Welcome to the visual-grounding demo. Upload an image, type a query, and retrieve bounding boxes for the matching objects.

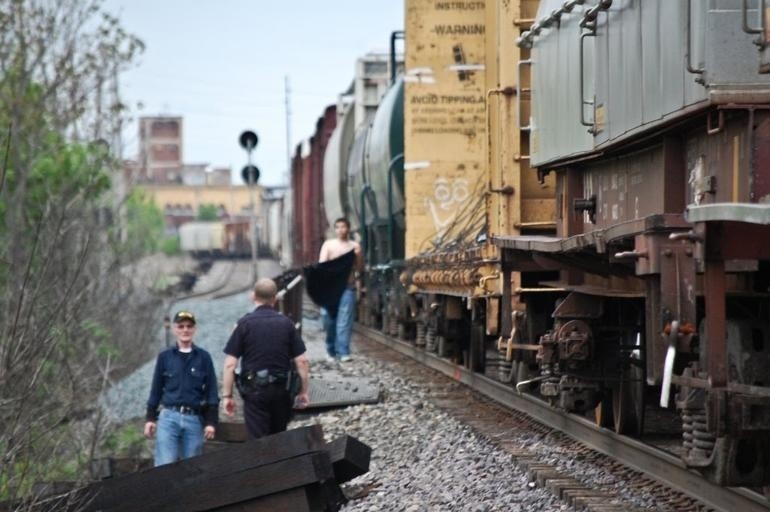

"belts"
[168,405,198,415]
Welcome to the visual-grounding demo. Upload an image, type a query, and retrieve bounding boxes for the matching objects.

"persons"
[317,217,362,363]
[221,277,310,441]
[144,311,220,468]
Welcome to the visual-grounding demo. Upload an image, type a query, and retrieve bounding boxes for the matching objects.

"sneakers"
[341,356,353,361]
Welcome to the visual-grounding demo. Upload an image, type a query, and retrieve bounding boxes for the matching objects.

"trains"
[177,0,769,486]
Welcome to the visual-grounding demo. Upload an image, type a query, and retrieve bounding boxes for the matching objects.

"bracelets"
[222,395,234,398]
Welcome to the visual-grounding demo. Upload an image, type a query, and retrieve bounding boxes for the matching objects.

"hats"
[173,311,195,324]
